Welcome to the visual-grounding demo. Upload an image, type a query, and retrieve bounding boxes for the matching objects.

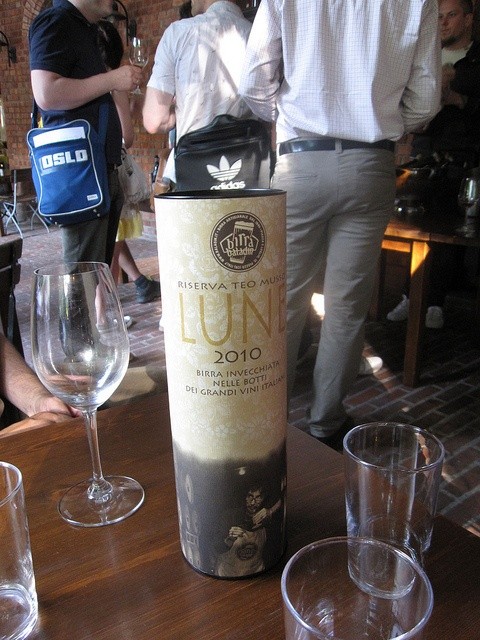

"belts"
[280,139,390,156]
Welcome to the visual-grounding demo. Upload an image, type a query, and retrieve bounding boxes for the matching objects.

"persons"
[27,0,146,372]
[385,1,478,332]
[0,321,84,438]
[142,0,272,189]
[239,1,443,447]
[111,240,160,304]
[90,20,146,335]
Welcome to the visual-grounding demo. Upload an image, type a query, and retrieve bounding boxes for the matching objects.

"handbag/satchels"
[394,152,466,233]
[173,114,271,191]
[26,8,112,227]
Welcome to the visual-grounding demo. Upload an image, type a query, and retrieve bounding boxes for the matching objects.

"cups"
[282,536,435,640]
[342,422,445,598]
[1,461,41,639]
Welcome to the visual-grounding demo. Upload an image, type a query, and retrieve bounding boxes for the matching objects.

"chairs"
[1,167,51,240]
[0,175,19,223]
[1,237,26,373]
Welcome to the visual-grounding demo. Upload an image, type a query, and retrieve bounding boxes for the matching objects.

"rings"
[136,79,141,85]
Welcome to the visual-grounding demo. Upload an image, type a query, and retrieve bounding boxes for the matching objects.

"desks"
[1,389,480,640]
[383,215,480,385]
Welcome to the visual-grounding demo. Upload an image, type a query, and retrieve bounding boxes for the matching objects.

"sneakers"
[359,346,383,376]
[386,294,410,321]
[96,316,133,334]
[136,280,160,304]
[426,306,444,329]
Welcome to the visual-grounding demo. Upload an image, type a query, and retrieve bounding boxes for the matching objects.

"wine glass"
[128,36,151,96]
[31,260,146,529]
[455,176,480,238]
